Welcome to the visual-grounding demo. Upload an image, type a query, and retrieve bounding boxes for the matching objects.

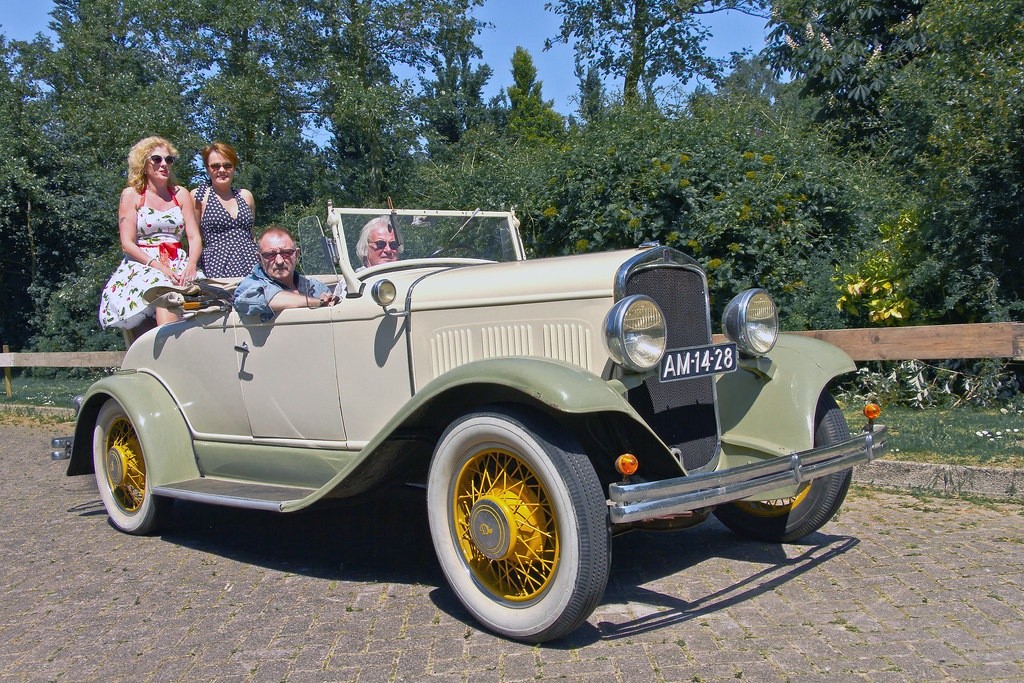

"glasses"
[369,240,399,250]
[146,155,176,164]
[260,249,295,259]
[208,162,234,170]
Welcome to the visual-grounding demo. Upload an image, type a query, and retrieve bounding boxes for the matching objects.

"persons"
[98,135,208,327]
[232,226,335,322]
[188,142,261,280]
[333,216,404,305]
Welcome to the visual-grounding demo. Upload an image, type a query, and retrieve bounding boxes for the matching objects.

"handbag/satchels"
[142,276,245,316]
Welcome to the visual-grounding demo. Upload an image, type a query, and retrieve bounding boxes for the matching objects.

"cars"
[50,199,898,644]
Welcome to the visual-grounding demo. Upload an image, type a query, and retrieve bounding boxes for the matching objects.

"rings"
[171,277,176,282]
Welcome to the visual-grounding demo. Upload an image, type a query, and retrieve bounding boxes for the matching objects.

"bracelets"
[145,258,157,269]
[317,297,326,308]
